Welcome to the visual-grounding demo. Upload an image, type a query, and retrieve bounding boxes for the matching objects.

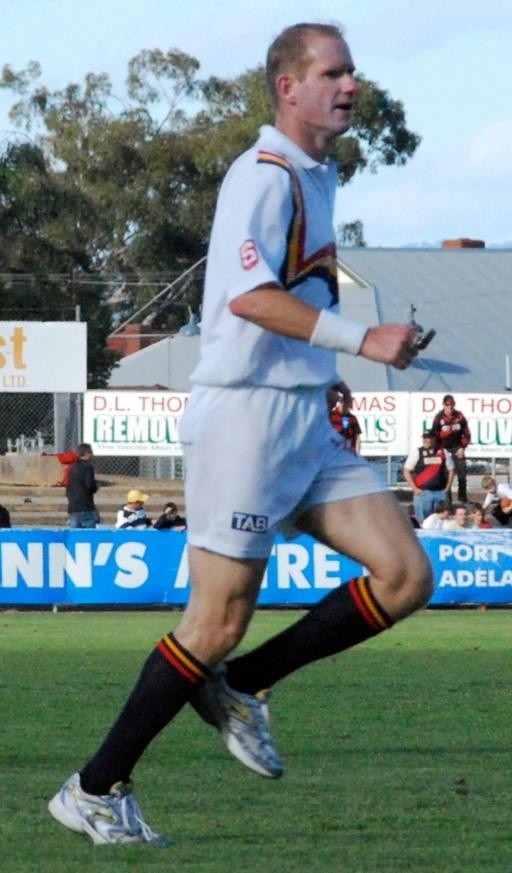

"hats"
[127,490,149,503]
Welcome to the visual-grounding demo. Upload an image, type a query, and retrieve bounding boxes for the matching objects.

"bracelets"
[306,305,371,358]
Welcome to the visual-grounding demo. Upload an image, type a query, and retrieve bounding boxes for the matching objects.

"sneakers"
[48,773,166,845]
[188,661,283,778]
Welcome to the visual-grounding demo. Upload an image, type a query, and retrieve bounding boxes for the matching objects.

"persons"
[421,499,493,529]
[430,395,471,504]
[480,476,512,526]
[115,490,154,530]
[329,394,362,454]
[404,430,456,524]
[45,21,434,850]
[65,445,100,530]
[154,502,187,531]
